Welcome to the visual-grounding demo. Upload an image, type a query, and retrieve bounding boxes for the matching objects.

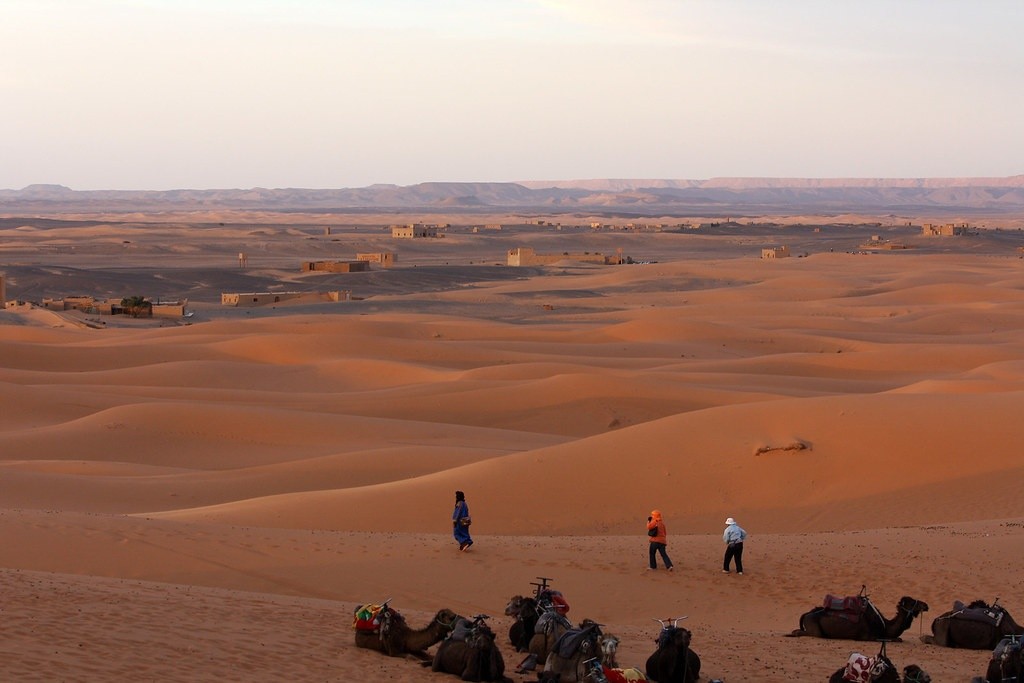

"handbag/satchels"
[647,520,658,536]
[460,517,471,526]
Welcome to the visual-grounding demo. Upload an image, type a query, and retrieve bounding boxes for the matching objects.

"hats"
[725,518,735,524]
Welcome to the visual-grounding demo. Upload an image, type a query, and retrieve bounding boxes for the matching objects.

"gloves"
[648,516,652,521]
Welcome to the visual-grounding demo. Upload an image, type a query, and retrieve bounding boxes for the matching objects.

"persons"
[647,510,673,572]
[722,518,747,575]
[452,491,473,551]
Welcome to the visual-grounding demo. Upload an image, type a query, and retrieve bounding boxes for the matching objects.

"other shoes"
[465,541,473,551]
[667,566,673,571]
[722,570,729,573]
[647,568,656,570]
[736,572,742,575]
[459,542,468,552]
